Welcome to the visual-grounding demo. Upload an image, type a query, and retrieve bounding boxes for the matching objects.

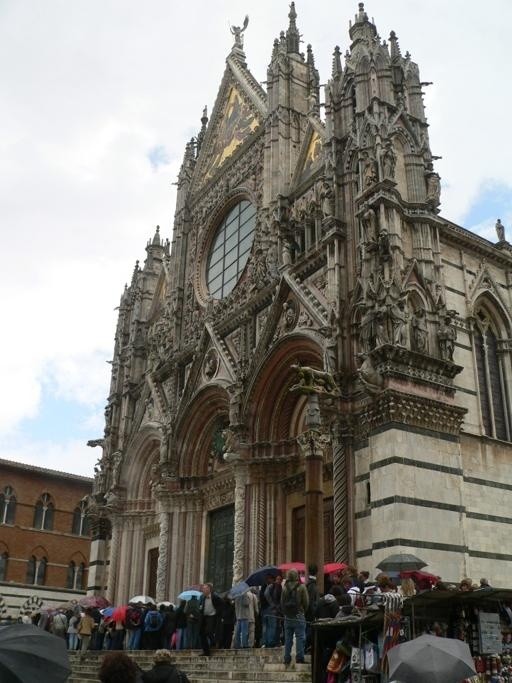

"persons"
[373,301,387,346]
[496,218,505,241]
[378,138,397,180]
[390,301,408,346]
[412,306,429,352]
[437,316,455,362]
[281,239,293,265]
[319,183,335,218]
[358,149,377,184]
[362,201,375,242]
[0,561,493,682]
[422,160,442,202]
[282,303,295,330]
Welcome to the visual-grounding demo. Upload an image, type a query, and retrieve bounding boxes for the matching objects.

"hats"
[362,585,376,595]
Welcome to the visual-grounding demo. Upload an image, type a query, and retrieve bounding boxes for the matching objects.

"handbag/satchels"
[128,610,143,626]
[175,612,187,629]
[326,639,379,683]
[355,591,366,608]
[186,606,200,621]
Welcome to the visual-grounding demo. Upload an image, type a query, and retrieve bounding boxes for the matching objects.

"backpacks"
[149,613,160,628]
[282,583,300,616]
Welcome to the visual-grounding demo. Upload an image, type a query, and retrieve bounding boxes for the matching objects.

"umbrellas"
[375,550,429,575]
